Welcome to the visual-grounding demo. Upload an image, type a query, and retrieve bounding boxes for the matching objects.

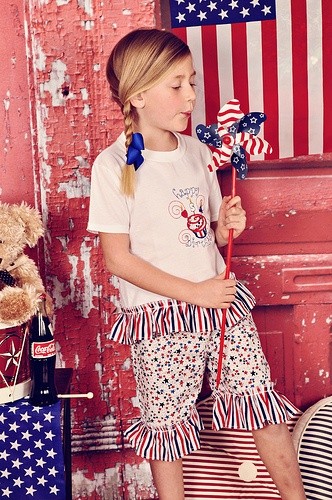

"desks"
[0,368,74,500]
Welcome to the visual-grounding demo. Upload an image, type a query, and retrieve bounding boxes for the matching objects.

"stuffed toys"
[0,202,56,325]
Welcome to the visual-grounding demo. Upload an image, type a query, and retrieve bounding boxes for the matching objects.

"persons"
[84,25,307,499]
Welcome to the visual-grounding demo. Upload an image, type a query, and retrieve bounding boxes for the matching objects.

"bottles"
[29,293,58,406]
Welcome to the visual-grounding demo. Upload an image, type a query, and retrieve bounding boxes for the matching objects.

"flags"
[0,397,67,500]
[169,0,332,171]
